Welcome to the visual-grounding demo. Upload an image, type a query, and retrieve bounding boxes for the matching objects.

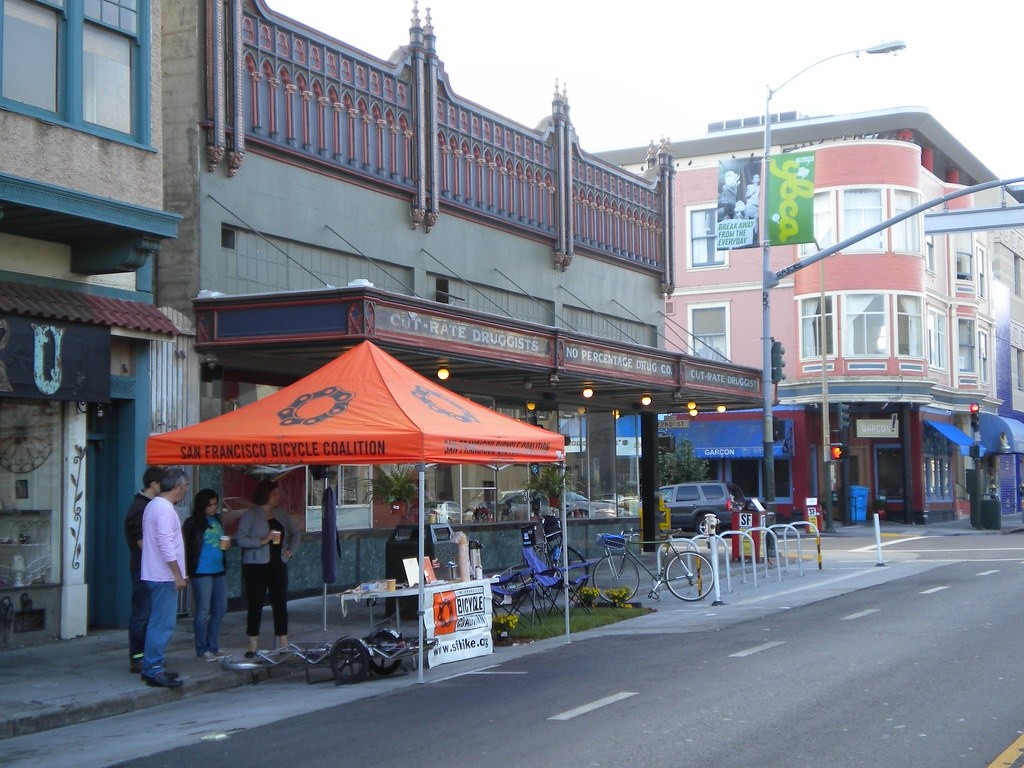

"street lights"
[772,342,786,385]
[970,403,980,431]
[762,39,907,555]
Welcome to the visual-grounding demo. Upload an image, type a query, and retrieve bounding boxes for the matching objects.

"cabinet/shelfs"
[0,507,54,591]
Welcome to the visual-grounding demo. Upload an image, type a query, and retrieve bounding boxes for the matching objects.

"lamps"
[524,380,533,390]
[717,403,726,413]
[641,395,651,405]
[526,400,621,419]
[437,367,451,379]
[687,401,699,416]
[583,388,593,397]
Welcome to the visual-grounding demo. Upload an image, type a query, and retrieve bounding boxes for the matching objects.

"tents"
[145,340,571,684]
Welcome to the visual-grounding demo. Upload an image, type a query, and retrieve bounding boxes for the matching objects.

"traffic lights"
[830,444,843,460]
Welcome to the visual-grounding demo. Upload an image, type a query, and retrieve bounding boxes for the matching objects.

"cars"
[409,501,475,524]
[497,489,641,519]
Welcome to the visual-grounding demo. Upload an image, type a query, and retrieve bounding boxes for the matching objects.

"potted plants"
[361,466,423,514]
[523,465,567,506]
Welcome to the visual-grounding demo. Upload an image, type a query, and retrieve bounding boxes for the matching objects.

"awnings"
[923,419,986,458]
[978,412,1024,453]
[560,413,645,459]
[659,419,795,460]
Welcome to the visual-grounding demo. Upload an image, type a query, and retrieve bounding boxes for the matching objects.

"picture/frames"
[15,478,28,500]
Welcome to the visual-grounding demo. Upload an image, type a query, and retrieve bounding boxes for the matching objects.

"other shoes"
[245,650,255,659]
[214,649,232,659]
[131,663,142,673]
[198,651,218,662]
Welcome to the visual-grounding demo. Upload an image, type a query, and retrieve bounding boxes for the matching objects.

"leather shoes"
[141,670,179,681]
[146,673,184,687]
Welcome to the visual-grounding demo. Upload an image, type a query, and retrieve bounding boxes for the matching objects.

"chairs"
[521,523,595,618]
[482,567,550,624]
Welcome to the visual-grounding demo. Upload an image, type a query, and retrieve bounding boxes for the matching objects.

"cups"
[271,530,281,544]
[387,579,396,591]
[430,513,436,523]
[475,565,482,580]
[457,534,471,583]
[219,536,229,550]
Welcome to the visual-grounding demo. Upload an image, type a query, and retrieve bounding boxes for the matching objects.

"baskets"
[597,532,626,549]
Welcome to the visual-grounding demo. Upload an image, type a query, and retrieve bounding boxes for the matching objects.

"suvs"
[658,482,747,536]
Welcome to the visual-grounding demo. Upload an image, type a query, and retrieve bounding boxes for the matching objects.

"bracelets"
[285,550,293,559]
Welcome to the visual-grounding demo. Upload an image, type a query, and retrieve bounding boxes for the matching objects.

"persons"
[182,488,232,663]
[237,480,300,658]
[126,464,168,674]
[141,468,188,687]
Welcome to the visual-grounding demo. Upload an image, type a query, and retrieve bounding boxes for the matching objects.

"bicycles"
[592,527,718,602]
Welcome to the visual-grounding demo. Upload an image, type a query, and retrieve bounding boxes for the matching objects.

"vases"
[614,597,628,607]
[496,626,512,639]
[582,596,593,606]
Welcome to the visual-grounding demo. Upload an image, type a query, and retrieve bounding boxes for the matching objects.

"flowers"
[602,586,633,600]
[492,613,519,630]
[580,587,600,600]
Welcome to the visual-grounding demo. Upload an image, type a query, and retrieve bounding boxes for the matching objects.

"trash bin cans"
[850,485,869,521]
[970,493,1001,529]
[385,523,459,615]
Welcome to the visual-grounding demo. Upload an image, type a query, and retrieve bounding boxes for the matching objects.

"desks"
[340,576,501,670]
